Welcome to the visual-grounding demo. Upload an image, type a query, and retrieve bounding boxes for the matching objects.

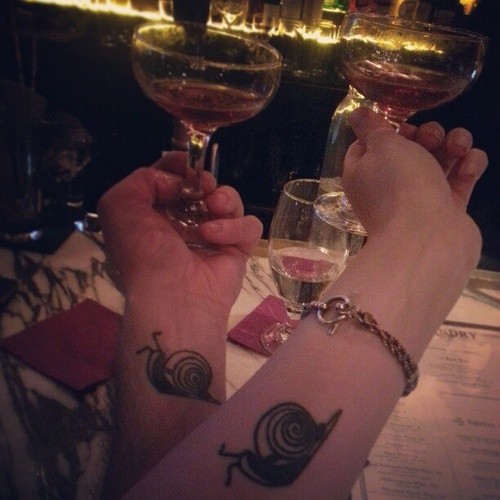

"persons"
[98,151,265,500]
[121,104,489,500]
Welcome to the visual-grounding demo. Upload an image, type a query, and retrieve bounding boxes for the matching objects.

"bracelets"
[300,292,419,398]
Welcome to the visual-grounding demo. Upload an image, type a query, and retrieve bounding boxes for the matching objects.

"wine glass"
[217,3,243,31]
[131,23,281,250]
[261,180,345,355]
[314,14,484,237]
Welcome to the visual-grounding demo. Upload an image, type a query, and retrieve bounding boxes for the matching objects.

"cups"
[253,14,339,78]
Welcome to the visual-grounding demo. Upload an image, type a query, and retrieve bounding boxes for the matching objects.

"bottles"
[313,84,407,236]
